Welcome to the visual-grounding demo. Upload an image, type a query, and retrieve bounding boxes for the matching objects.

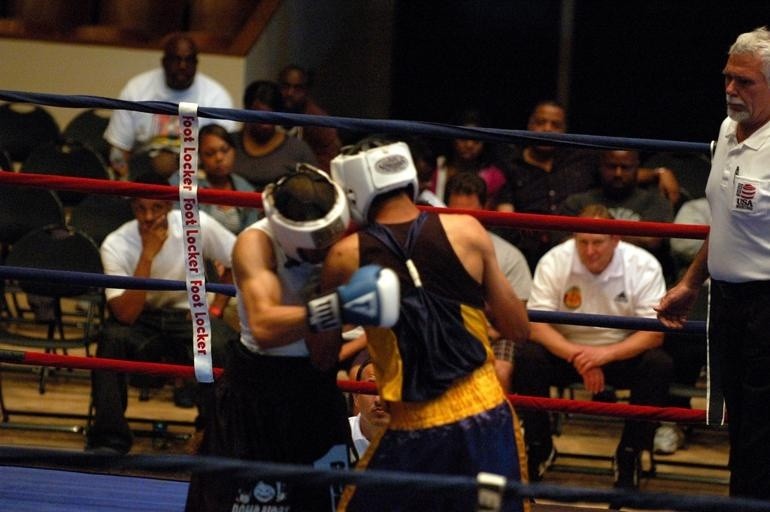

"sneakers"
[87,430,133,458]
[652,419,687,456]
[527,444,558,483]
[612,441,641,491]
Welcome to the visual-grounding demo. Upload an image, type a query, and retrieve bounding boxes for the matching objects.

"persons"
[83,34,709,511]
[651,24,770,511]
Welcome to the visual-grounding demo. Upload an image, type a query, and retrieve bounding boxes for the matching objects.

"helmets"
[259,162,353,271]
[328,132,421,228]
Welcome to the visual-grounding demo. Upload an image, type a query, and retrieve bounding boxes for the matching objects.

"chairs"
[1,96,705,449]
